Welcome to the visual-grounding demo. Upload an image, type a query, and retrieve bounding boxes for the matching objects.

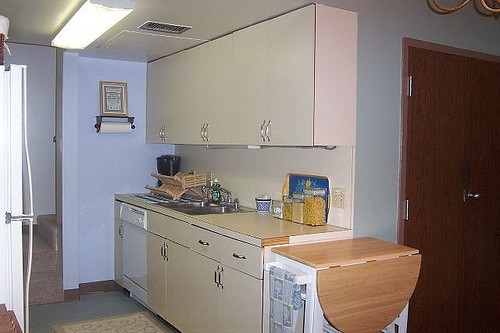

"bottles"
[156,155,181,186]
[201,185,212,202]
[212,178,221,204]
[271,188,328,229]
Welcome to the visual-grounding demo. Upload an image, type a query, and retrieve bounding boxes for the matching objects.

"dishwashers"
[118,203,147,304]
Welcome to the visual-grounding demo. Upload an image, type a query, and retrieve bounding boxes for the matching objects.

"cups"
[255,197,271,214]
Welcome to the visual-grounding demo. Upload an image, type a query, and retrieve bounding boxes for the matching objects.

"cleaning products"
[208,178,221,204]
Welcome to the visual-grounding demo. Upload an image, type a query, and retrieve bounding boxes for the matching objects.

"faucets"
[209,185,232,203]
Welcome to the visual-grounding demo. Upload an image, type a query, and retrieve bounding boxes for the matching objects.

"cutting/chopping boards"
[282,173,330,227]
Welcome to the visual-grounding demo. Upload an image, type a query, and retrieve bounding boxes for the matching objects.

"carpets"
[52,311,170,333]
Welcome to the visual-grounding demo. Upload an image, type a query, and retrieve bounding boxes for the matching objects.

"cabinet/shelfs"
[146,2,357,147]
[113,193,354,333]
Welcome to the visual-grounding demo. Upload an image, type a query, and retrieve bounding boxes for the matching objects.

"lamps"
[50,0,135,49]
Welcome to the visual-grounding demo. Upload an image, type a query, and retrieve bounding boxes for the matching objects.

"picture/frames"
[99,81,128,117]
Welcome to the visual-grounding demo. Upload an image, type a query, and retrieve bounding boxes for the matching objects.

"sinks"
[171,205,240,215]
[152,199,228,209]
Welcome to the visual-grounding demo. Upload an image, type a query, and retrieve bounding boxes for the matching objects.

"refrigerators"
[0,63,34,333]
